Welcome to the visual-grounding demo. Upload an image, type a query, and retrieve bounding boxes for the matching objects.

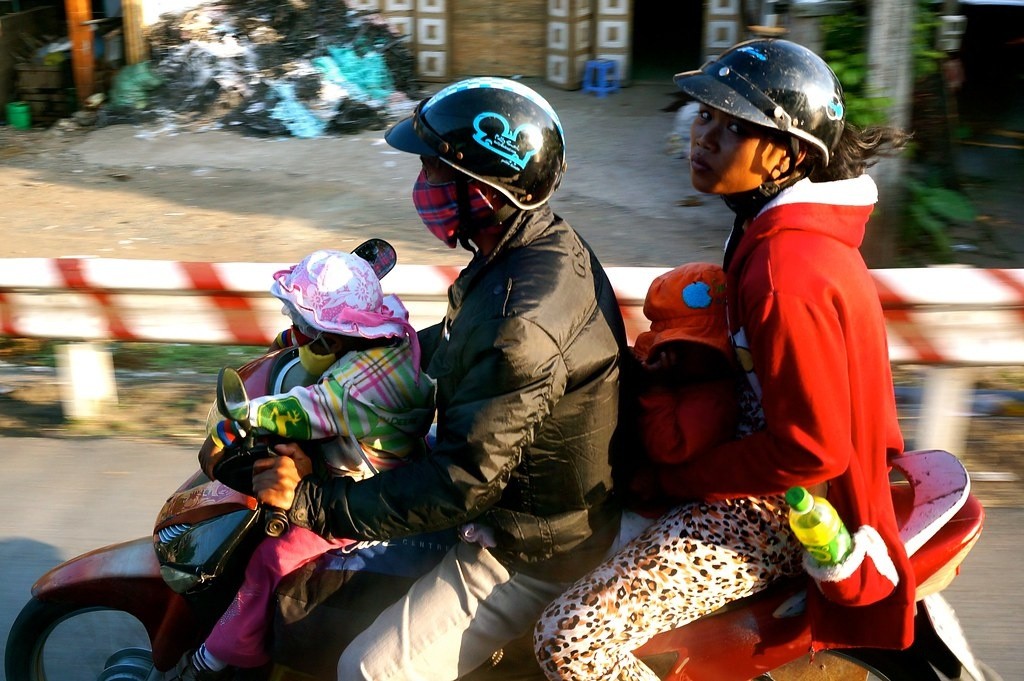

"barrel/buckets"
[7,102,29,130]
[16,63,69,123]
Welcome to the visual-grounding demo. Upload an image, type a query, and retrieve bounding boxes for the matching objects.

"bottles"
[786,487,851,566]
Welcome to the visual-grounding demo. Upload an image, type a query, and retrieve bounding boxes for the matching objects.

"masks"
[412,169,498,249]
[299,338,335,378]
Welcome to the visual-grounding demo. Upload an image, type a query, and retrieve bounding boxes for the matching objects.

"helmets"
[672,41,847,171]
[383,77,568,213]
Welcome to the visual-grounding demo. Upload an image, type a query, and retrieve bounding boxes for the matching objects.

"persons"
[162,250,436,681]
[252,76,634,681]
[533,38,915,681]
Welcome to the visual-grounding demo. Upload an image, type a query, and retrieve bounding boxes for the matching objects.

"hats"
[639,263,735,372]
[269,249,424,388]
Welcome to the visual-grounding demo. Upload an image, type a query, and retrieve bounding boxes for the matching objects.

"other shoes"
[148,650,235,681]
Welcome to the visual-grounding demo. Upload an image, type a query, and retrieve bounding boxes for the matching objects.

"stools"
[582,59,619,99]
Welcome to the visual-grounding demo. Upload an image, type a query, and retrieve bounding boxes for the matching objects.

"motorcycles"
[4,238,986,681]
